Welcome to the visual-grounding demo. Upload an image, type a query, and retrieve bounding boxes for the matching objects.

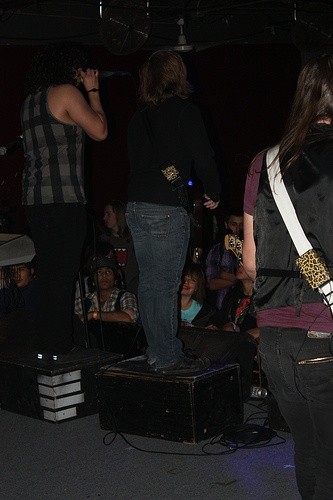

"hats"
[91,257,117,273]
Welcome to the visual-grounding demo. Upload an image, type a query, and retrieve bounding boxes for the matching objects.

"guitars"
[190,192,203,233]
[224,233,244,257]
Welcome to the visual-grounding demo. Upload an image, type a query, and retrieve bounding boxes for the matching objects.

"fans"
[133,17,248,52]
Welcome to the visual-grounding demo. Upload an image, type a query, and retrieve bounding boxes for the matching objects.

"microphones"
[84,70,128,78]
[0,134,23,156]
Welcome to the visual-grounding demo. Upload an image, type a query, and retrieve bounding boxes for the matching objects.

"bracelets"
[183,321,188,327]
[87,88,100,93]
[92,310,98,321]
[229,322,237,332]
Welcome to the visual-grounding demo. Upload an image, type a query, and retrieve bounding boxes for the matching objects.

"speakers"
[177,326,247,361]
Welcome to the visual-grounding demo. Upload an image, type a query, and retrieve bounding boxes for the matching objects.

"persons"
[97,200,139,297]
[242,54,333,500]
[74,258,138,323]
[75,241,125,298]
[205,264,260,381]
[206,206,244,311]
[19,43,108,365]
[125,49,223,376]
[0,263,36,314]
[176,263,220,331]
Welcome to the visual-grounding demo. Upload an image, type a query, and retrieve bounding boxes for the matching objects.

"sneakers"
[146,349,210,375]
[39,346,100,363]
